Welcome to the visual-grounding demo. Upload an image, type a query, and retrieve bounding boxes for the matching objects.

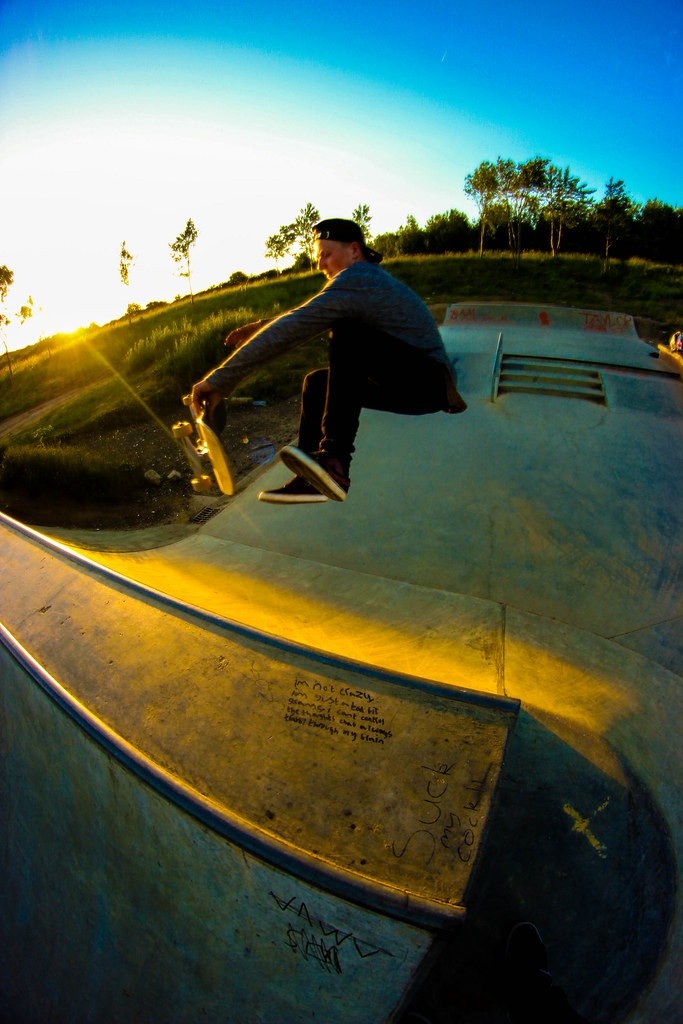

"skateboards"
[172,394,236,496]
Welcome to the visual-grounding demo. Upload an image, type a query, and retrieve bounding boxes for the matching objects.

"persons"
[189,217,468,505]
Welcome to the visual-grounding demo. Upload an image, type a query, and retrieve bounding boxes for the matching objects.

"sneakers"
[281,445,351,502]
[258,474,328,504]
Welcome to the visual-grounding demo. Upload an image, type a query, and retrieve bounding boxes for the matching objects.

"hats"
[312,218,383,264]
